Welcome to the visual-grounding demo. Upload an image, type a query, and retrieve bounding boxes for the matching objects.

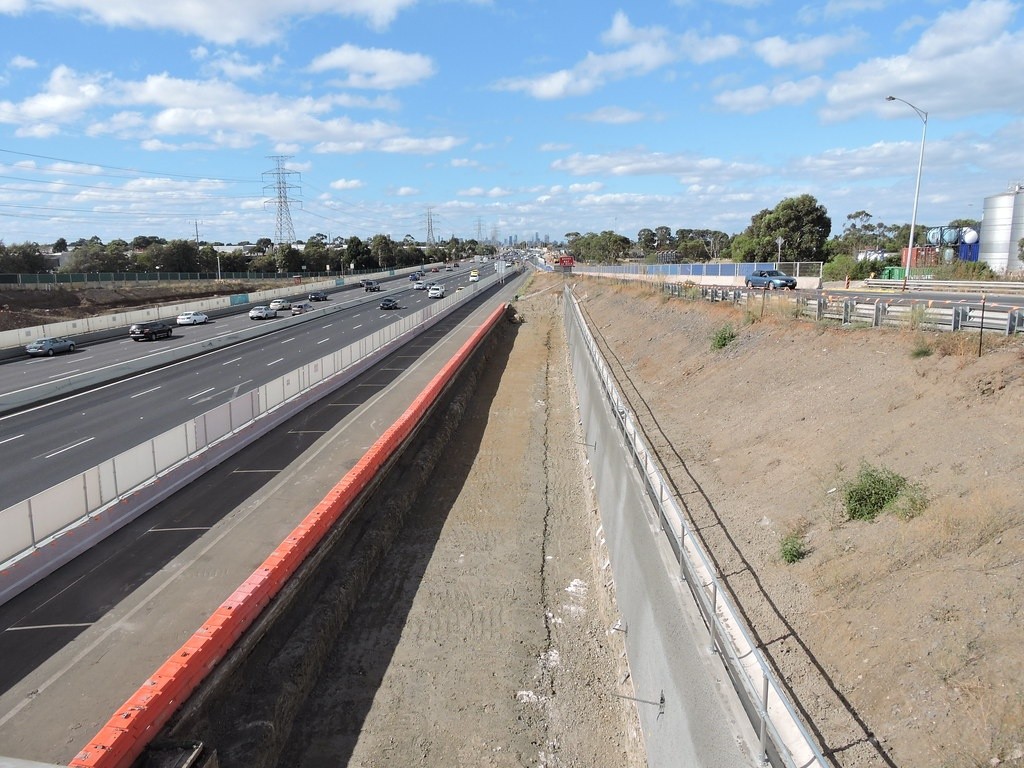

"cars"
[469,273,480,282]
[249,305,277,320]
[470,269,480,276]
[408,270,443,299]
[453,262,460,267]
[455,287,466,293]
[445,265,454,271]
[431,267,439,272]
[269,298,293,311]
[470,245,567,272]
[379,297,400,310]
[176,310,209,326]
[26,336,76,357]
[308,291,327,301]
[291,303,315,316]
[744,269,797,291]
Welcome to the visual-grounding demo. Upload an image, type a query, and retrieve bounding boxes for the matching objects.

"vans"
[360,279,369,287]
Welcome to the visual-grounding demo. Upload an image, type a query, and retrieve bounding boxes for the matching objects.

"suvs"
[128,320,173,342]
[364,280,381,292]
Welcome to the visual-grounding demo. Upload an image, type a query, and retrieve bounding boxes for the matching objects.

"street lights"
[884,94,929,280]
